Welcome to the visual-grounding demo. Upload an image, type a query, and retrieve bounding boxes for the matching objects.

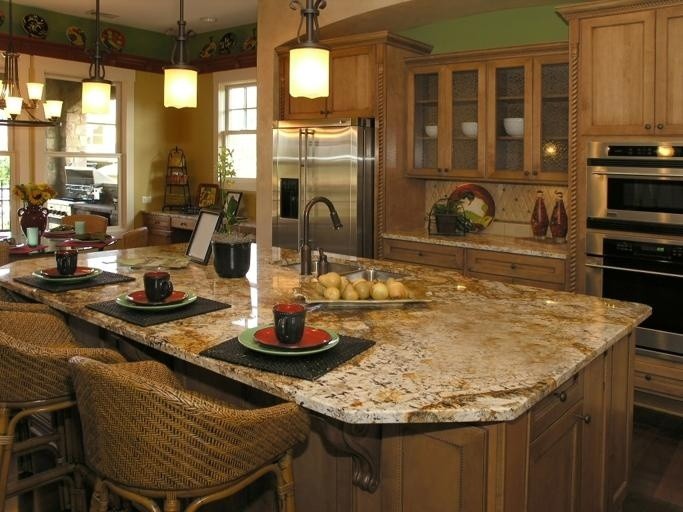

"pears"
[389,282,404,299]
[322,287,340,300]
[339,276,349,297]
[318,272,341,289]
[352,278,366,287]
[355,280,369,299]
[386,277,395,288]
[372,283,389,300]
[342,282,359,300]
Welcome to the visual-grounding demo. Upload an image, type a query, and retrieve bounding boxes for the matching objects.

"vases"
[17,205,49,244]
[218,189,226,208]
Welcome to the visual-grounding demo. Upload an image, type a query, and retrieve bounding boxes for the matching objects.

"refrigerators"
[273,115,374,260]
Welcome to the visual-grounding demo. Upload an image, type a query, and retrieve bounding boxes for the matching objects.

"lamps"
[0,52,64,126]
[289,0,330,99]
[162,0,199,109]
[82,0,112,114]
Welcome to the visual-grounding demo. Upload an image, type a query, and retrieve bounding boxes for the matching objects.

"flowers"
[215,147,235,192]
[11,182,58,205]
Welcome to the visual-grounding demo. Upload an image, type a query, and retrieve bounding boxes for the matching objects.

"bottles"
[530,190,549,240]
[550,192,568,239]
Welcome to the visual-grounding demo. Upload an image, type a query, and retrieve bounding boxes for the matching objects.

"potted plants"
[434,202,459,234]
[212,196,251,278]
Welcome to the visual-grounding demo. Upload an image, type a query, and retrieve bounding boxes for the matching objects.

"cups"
[143,270,173,303]
[55,249,78,275]
[73,221,86,236]
[27,227,40,248]
[270,303,306,344]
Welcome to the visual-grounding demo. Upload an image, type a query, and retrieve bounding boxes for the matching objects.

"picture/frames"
[196,183,219,208]
[185,210,222,266]
[221,191,243,216]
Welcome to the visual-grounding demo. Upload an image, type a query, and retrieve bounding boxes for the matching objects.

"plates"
[115,289,198,311]
[32,267,103,282]
[251,326,332,351]
[293,276,434,306]
[126,290,189,306]
[237,323,342,356]
[41,266,94,278]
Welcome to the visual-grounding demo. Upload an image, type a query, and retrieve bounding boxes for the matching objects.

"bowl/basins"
[424,124,438,137]
[502,117,525,138]
[460,120,477,138]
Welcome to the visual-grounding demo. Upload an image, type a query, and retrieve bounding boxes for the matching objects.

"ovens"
[582,140,682,365]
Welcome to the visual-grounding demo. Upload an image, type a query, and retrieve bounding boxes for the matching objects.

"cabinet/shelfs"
[46,200,87,229]
[140,210,255,246]
[276,1,683,420]
[284,43,377,121]
[403,51,568,177]
[162,147,192,214]
[384,237,566,295]
[581,8,683,137]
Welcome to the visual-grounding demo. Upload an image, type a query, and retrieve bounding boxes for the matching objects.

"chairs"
[141,211,185,245]
[123,227,148,248]
[0,303,129,511]
[70,355,309,512]
[58,215,108,236]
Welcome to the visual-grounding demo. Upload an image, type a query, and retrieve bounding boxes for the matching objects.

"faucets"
[299,196,344,275]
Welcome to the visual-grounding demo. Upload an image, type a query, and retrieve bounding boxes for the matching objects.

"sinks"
[286,261,360,274]
[338,268,413,283]
